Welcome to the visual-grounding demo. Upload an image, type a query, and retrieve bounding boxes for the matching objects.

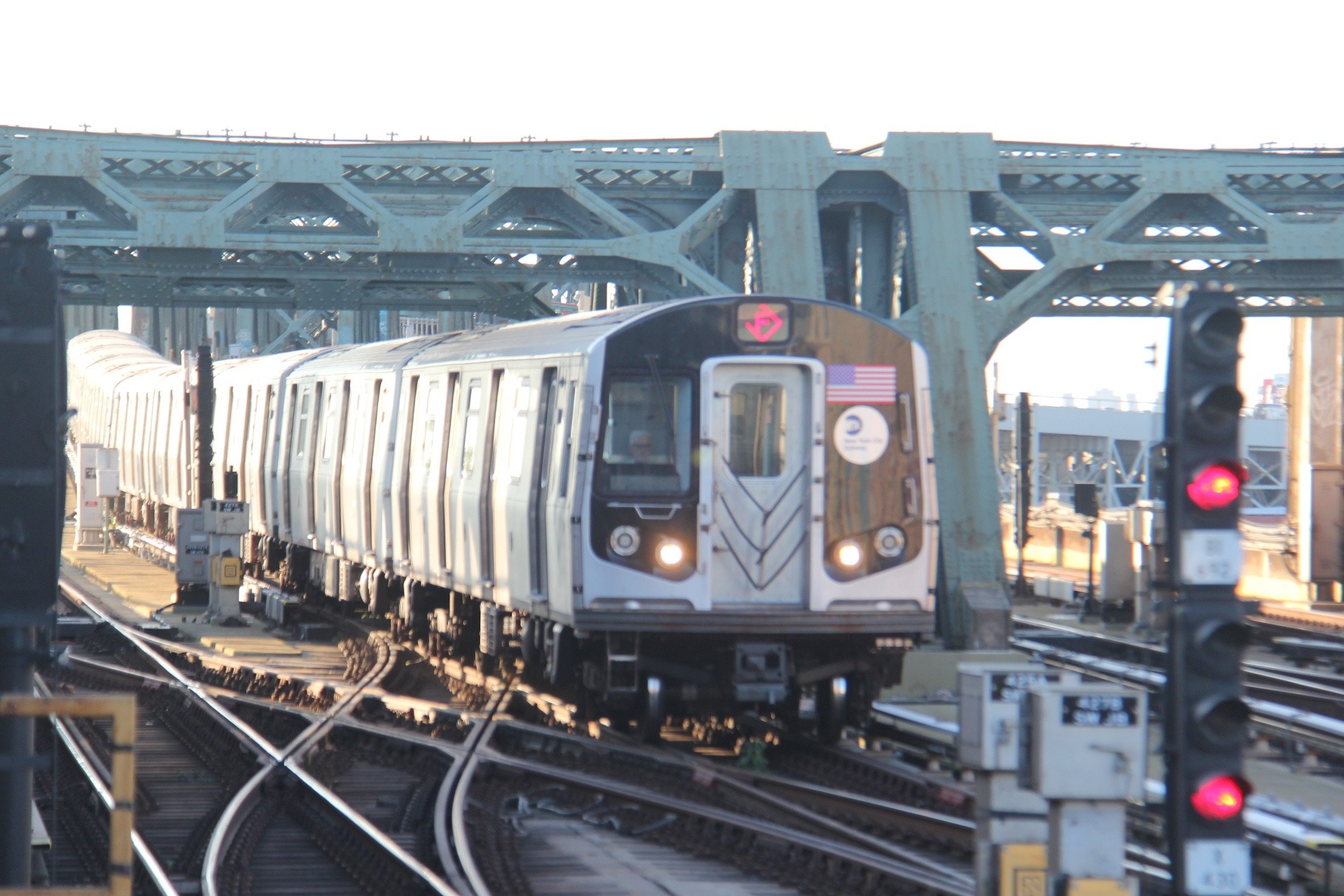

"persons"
[624,429,657,464]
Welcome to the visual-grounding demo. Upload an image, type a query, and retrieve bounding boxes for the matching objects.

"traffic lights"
[1163,600,1256,896]
[1164,290,1245,599]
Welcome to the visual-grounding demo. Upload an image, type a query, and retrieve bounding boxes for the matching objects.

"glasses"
[631,444,651,449]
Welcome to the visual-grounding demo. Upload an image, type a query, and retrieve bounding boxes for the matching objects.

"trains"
[68,293,941,743]
